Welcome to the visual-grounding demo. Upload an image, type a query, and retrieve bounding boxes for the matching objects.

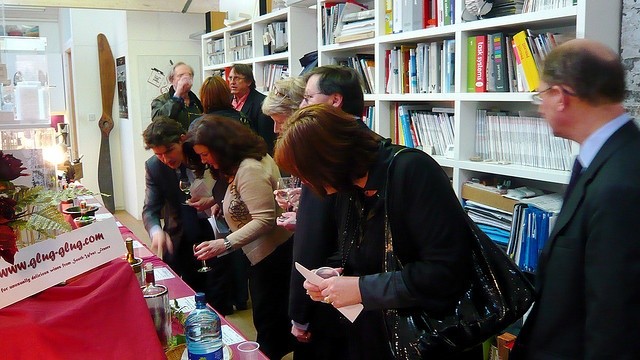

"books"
[322,3,373,45]
[464,2,576,19]
[359,105,371,133]
[329,53,373,90]
[202,71,224,98]
[261,21,285,57]
[382,2,459,35]
[469,106,585,169]
[259,64,291,90]
[460,179,568,278]
[466,33,570,93]
[384,39,455,92]
[229,29,253,60]
[392,107,453,155]
[204,39,225,64]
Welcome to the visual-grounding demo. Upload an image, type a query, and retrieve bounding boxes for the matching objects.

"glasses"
[227,75,245,80]
[304,91,322,104]
[532,86,551,105]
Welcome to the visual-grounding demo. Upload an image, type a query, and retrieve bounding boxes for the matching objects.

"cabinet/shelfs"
[315,1,587,359]
[201,5,294,123]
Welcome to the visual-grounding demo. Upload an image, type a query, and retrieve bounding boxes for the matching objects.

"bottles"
[124,237,143,285]
[138,261,172,351]
[181,292,224,359]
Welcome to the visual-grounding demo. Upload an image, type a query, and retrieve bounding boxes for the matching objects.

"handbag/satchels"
[381,147,535,359]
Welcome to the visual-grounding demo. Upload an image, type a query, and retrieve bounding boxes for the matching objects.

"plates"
[179,343,233,360]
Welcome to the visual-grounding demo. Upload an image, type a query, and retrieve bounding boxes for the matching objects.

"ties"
[560,157,582,213]
[179,167,188,179]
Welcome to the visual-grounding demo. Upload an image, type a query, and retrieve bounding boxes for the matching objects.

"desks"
[1,178,269,360]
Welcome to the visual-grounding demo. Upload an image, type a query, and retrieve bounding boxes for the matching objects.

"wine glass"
[278,177,295,222]
[193,244,211,273]
[180,182,193,206]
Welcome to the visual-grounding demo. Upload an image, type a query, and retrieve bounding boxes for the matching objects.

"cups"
[314,267,341,282]
[238,341,259,360]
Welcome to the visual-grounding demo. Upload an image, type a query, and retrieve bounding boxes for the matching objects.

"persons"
[226,64,274,135]
[510,39,635,358]
[290,66,369,352]
[192,115,293,356]
[142,117,245,311]
[261,78,306,137]
[153,64,201,117]
[202,76,245,116]
[273,105,540,360]
